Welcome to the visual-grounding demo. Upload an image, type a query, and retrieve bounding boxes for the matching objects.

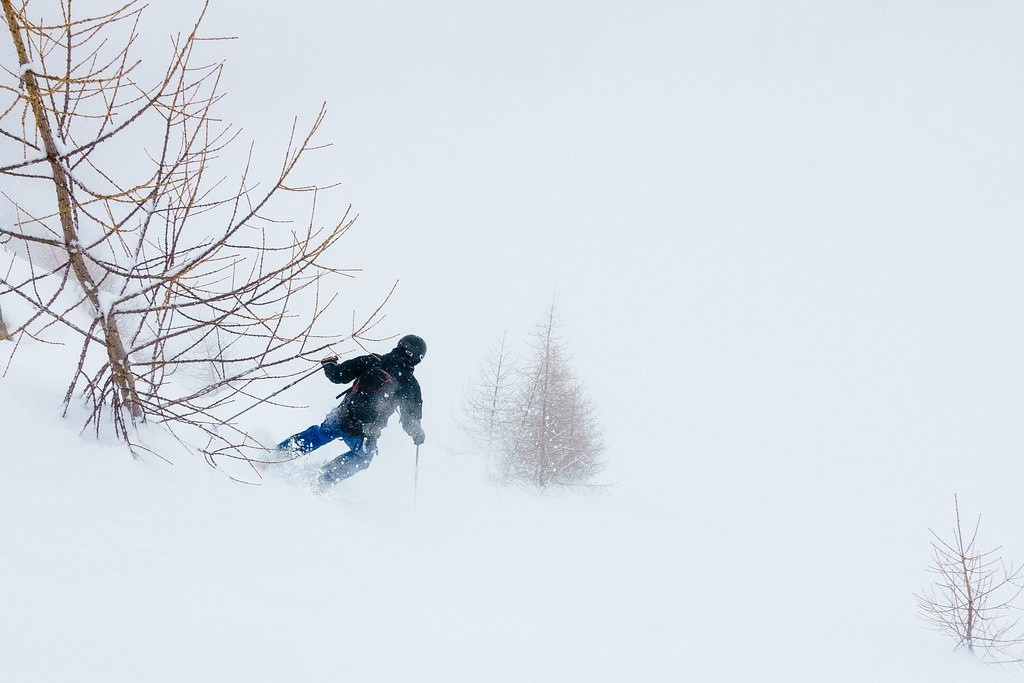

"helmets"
[397,334,427,365]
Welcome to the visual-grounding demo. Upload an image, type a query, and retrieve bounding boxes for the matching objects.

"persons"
[276,334,427,493]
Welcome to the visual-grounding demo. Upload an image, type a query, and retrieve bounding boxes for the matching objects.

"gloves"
[413,428,425,445]
[321,355,337,367]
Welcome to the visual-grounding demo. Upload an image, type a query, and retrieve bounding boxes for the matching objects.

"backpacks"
[337,366,401,439]
